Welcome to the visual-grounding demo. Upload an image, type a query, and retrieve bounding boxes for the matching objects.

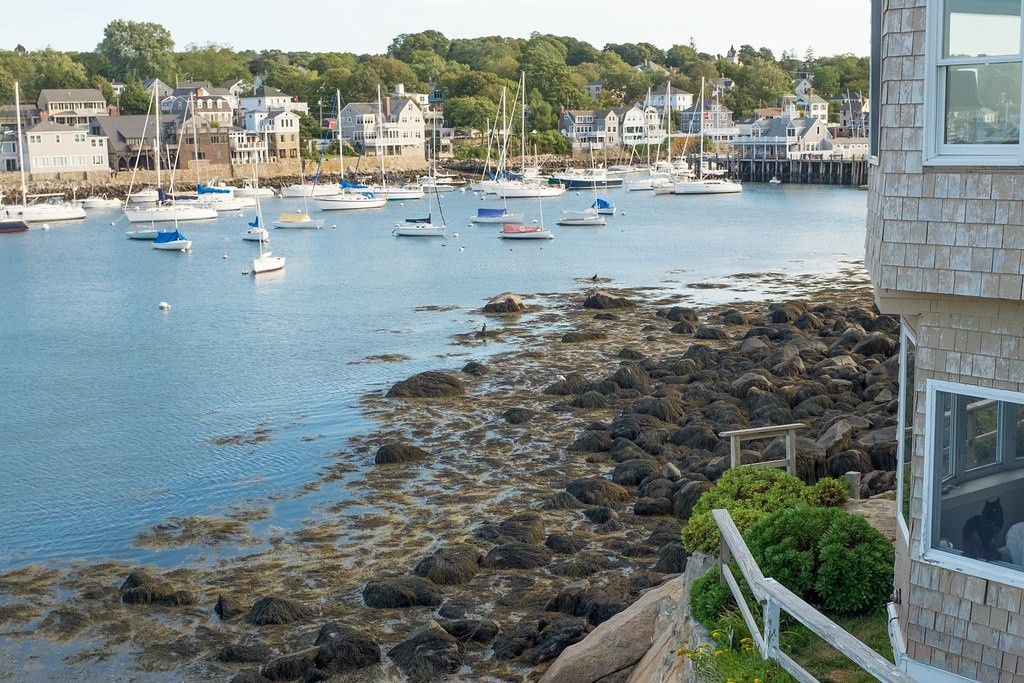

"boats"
[546,168,625,190]
[126,230,161,239]
[314,193,387,210]
[234,189,275,198]
[497,224,552,240]
[154,236,192,250]
[0,211,28,233]
[252,254,286,275]
[470,70,566,198]
[395,222,446,236]
[273,214,324,229]
[553,216,604,226]
[651,183,681,195]
[423,185,454,193]
[121,207,218,224]
[434,173,468,185]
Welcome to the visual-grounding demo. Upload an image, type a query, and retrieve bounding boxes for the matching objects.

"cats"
[960,496,1004,562]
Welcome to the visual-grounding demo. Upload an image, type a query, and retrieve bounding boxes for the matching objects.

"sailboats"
[675,76,743,196]
[82,80,162,209]
[624,84,670,192]
[343,83,424,200]
[242,199,270,242]
[1,81,87,222]
[166,89,256,213]
[280,88,341,200]
